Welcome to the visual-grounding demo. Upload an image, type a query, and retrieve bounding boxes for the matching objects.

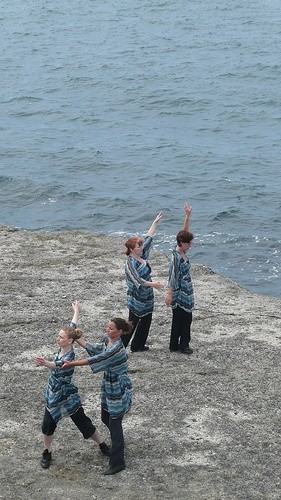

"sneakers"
[40,448,51,469]
[99,442,112,457]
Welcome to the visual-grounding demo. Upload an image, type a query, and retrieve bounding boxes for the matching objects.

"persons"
[60,317,134,475]
[33,300,115,469]
[120,211,166,351]
[164,202,195,355]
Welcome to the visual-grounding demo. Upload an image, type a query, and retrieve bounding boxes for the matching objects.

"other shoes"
[170,344,182,352]
[132,346,149,352]
[183,348,193,354]
[104,462,126,475]
